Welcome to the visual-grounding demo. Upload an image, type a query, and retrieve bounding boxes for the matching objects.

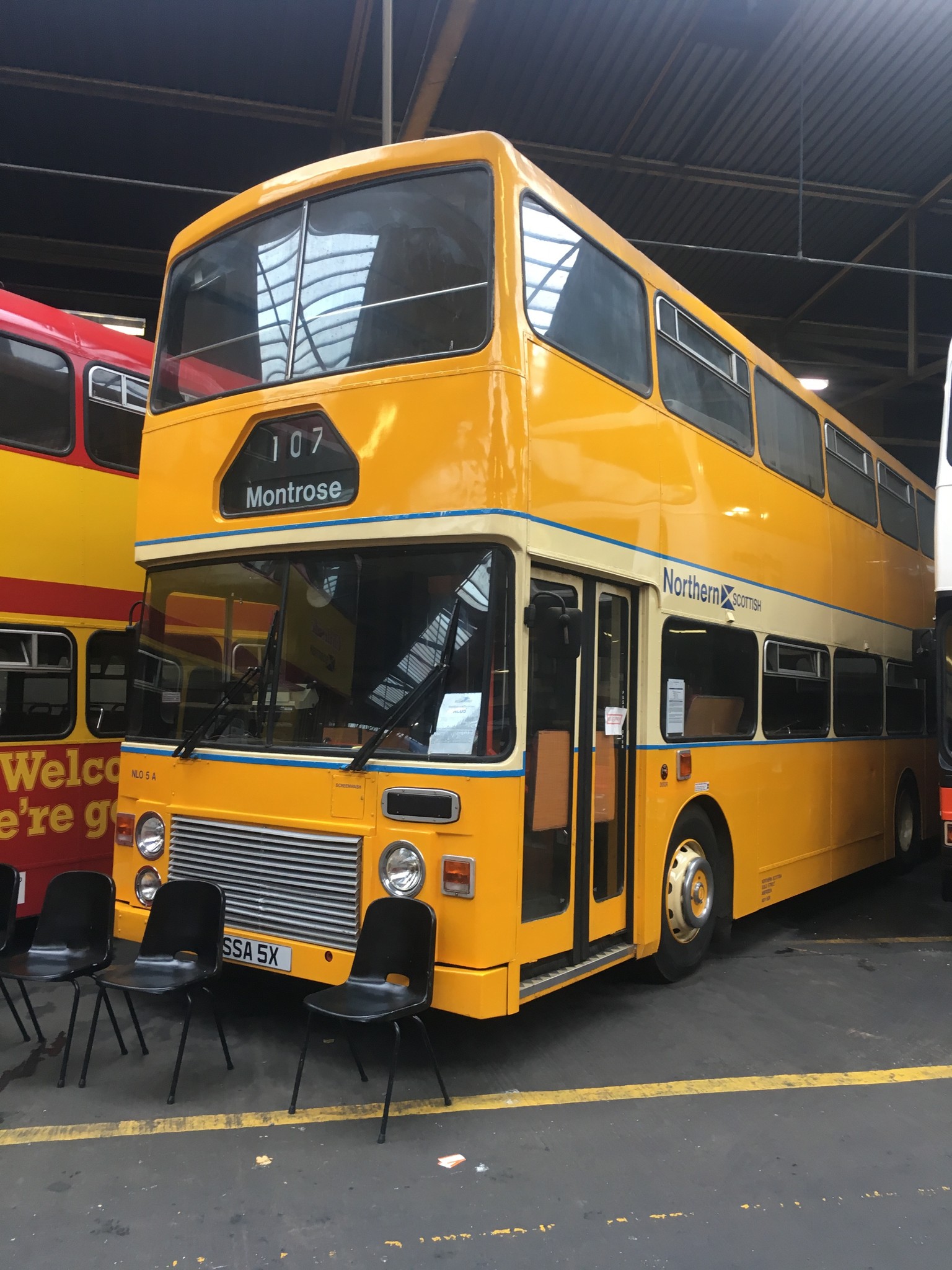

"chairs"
[288,897,451,1144]
[0,863,30,1042]
[0,870,128,1088]
[78,879,234,1105]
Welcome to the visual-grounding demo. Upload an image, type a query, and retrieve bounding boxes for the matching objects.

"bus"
[87,131,936,1025]
[0,289,309,963]
